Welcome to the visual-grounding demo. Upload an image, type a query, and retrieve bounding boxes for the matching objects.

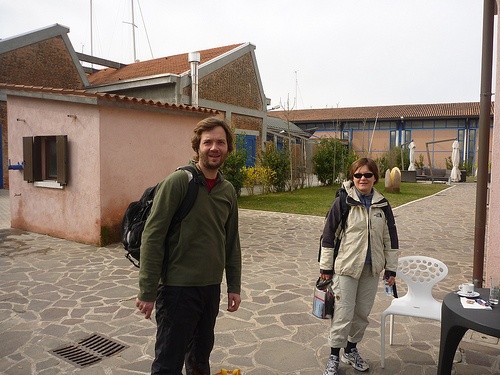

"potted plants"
[413,154,424,175]
[443,156,468,182]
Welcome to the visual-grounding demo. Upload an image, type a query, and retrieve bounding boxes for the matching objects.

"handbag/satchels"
[310,274,335,320]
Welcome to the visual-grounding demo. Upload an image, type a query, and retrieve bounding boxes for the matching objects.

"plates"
[457,291,480,297]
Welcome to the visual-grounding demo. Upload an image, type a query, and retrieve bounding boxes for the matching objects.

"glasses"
[353,172,374,178]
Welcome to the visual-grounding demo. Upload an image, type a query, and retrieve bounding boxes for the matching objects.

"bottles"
[384,277,393,296]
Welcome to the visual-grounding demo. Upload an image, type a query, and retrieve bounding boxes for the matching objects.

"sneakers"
[323,354,340,375]
[341,347,369,372]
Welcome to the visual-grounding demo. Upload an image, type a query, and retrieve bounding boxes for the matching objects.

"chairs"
[380,256,449,369]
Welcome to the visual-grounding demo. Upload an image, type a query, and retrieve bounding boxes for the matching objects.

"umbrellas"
[450,140,462,182]
[408,142,416,171]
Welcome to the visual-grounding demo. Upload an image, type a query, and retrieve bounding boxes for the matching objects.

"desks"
[437,288,500,375]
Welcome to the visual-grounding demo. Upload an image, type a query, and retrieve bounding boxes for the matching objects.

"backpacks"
[121,165,202,268]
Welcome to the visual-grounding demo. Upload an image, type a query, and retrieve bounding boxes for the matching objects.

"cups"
[489,278,500,304]
[459,284,474,296]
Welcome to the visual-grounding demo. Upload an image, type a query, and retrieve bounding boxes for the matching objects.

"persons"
[136,117,241,375]
[318,158,399,375]
